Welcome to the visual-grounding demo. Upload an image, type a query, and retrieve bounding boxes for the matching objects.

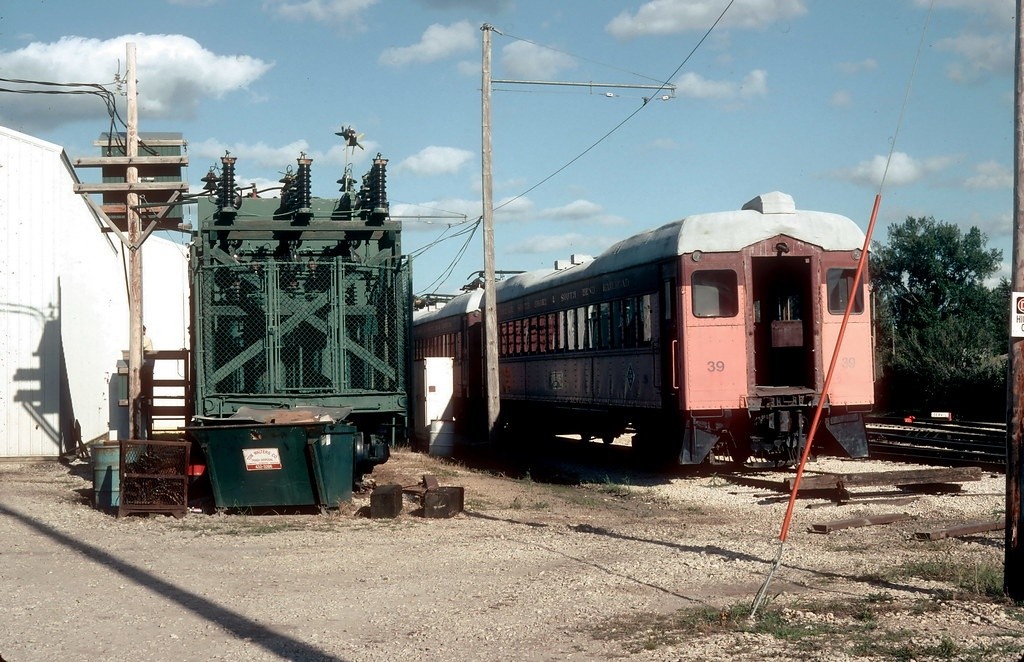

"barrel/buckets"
[428,419,456,460]
[94,443,145,511]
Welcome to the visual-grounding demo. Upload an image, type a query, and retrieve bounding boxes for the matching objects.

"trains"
[191,123,410,489]
[413,190,875,474]
